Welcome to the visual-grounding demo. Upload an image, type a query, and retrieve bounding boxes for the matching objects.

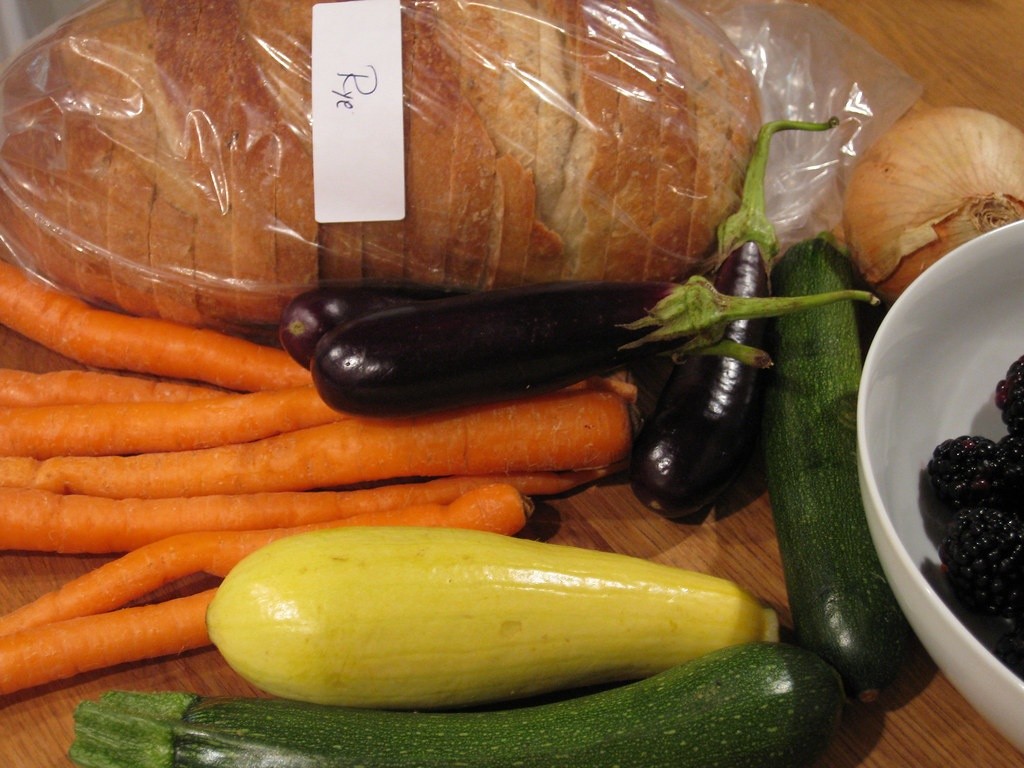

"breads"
[0,0,772,330]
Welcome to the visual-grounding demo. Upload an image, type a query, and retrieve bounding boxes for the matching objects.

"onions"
[842,101,1023,305]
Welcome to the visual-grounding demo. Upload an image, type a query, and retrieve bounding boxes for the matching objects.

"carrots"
[0,263,639,712]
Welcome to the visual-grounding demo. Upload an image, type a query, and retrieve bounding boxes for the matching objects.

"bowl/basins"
[853,210,1022,754]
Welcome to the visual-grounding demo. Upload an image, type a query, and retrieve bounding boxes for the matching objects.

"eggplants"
[630,112,842,521]
[311,277,882,418]
